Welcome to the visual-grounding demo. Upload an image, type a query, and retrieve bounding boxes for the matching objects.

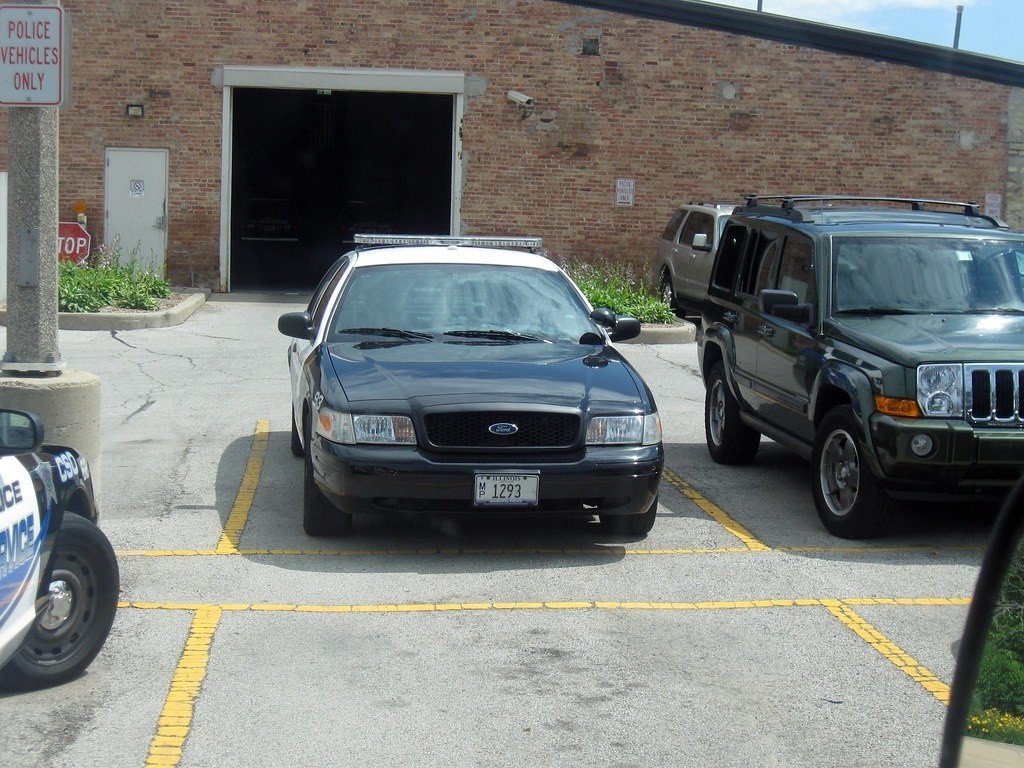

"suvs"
[653,202,748,319]
[694,193,1024,537]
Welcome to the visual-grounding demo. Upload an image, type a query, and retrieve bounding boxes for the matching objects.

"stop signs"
[58,221,91,266]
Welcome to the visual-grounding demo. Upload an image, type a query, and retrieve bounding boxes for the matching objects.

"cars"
[276,234,666,537]
[0,408,120,689]
